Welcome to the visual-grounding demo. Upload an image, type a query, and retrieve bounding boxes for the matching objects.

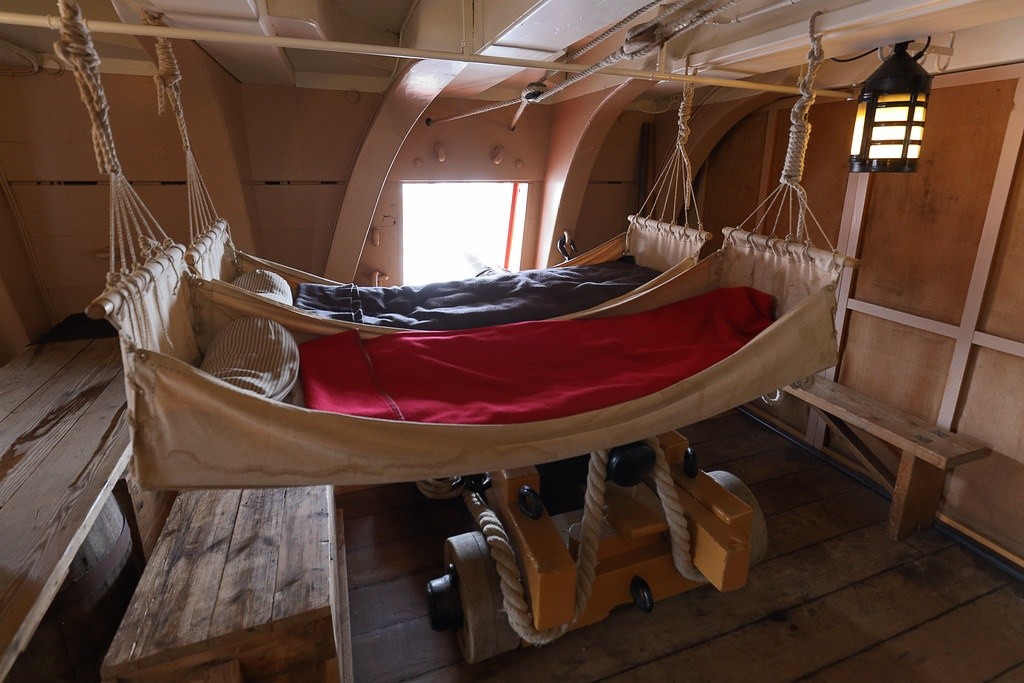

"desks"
[0,314,163,683]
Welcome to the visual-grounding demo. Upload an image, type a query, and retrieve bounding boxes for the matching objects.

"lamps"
[847,41,931,170]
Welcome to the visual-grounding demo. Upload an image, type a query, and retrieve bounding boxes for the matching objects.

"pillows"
[195,318,300,401]
[234,270,293,306]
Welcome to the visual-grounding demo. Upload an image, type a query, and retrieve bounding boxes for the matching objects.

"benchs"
[106,485,353,682]
[779,378,989,541]
